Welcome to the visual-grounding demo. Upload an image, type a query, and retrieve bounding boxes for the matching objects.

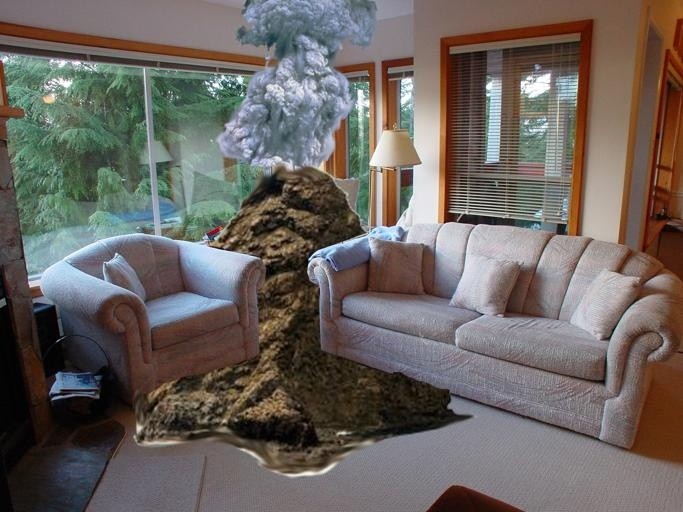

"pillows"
[367,237,426,295]
[448,252,525,318]
[569,268,643,341]
[102,251,147,302]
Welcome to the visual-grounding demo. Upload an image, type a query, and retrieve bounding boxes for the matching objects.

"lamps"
[368,122,422,233]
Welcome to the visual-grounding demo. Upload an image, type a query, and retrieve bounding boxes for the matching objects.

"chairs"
[39,233,265,409]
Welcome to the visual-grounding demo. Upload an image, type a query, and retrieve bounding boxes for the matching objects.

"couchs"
[308,223,683,450]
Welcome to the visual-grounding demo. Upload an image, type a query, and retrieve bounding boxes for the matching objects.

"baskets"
[44,335,111,418]
